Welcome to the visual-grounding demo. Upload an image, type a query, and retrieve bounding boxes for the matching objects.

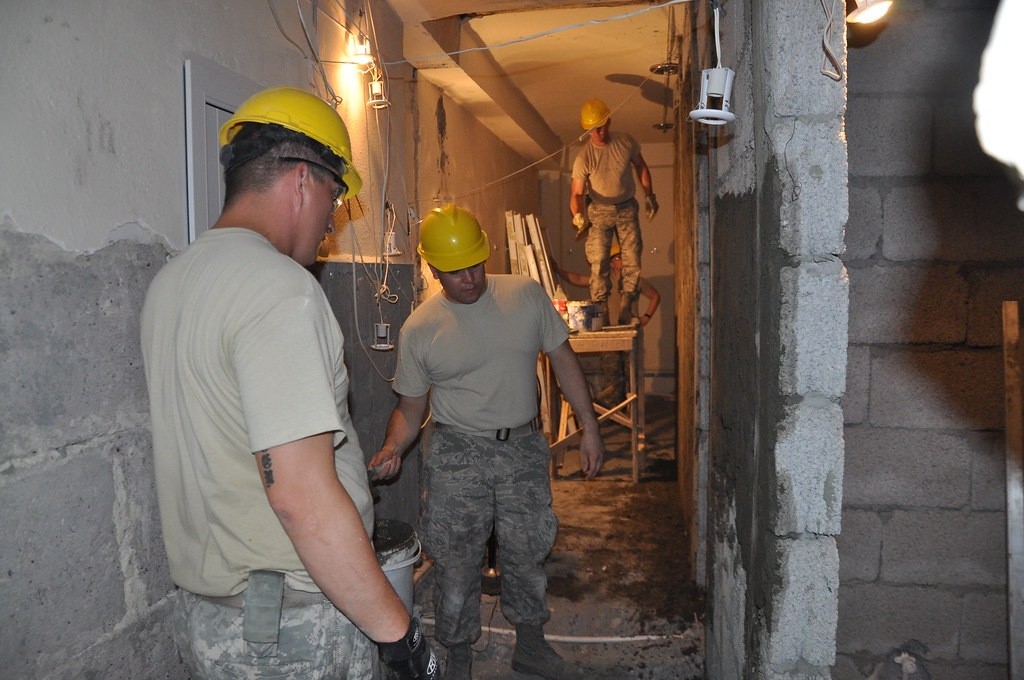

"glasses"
[611,256,622,262]
[279,158,349,207]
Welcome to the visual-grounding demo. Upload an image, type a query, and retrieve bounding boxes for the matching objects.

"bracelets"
[644,314,651,318]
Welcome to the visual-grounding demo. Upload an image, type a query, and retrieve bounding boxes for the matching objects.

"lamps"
[382,232,402,257]
[370,322,394,351]
[689,70,736,125]
[846,0,895,23]
[352,36,373,65]
[366,80,391,110]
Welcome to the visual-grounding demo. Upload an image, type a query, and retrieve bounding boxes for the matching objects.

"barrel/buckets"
[372,520,421,619]
[567,301,602,332]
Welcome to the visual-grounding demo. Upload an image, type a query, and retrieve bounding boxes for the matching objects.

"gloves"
[378,614,440,680]
[644,194,659,223]
[571,213,588,229]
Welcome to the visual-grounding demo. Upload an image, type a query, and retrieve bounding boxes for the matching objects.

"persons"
[140,87,660,680]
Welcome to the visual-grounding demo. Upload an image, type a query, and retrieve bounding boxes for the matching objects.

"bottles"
[552,284,568,324]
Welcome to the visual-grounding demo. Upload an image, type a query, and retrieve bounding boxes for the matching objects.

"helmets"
[609,243,621,259]
[218,86,363,199]
[581,99,610,129]
[417,203,491,272]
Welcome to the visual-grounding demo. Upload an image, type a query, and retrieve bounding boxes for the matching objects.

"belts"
[434,418,540,441]
[194,590,329,608]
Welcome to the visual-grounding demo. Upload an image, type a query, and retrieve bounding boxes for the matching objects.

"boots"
[442,641,473,679]
[510,623,584,680]
[592,301,610,331]
[616,294,640,325]
